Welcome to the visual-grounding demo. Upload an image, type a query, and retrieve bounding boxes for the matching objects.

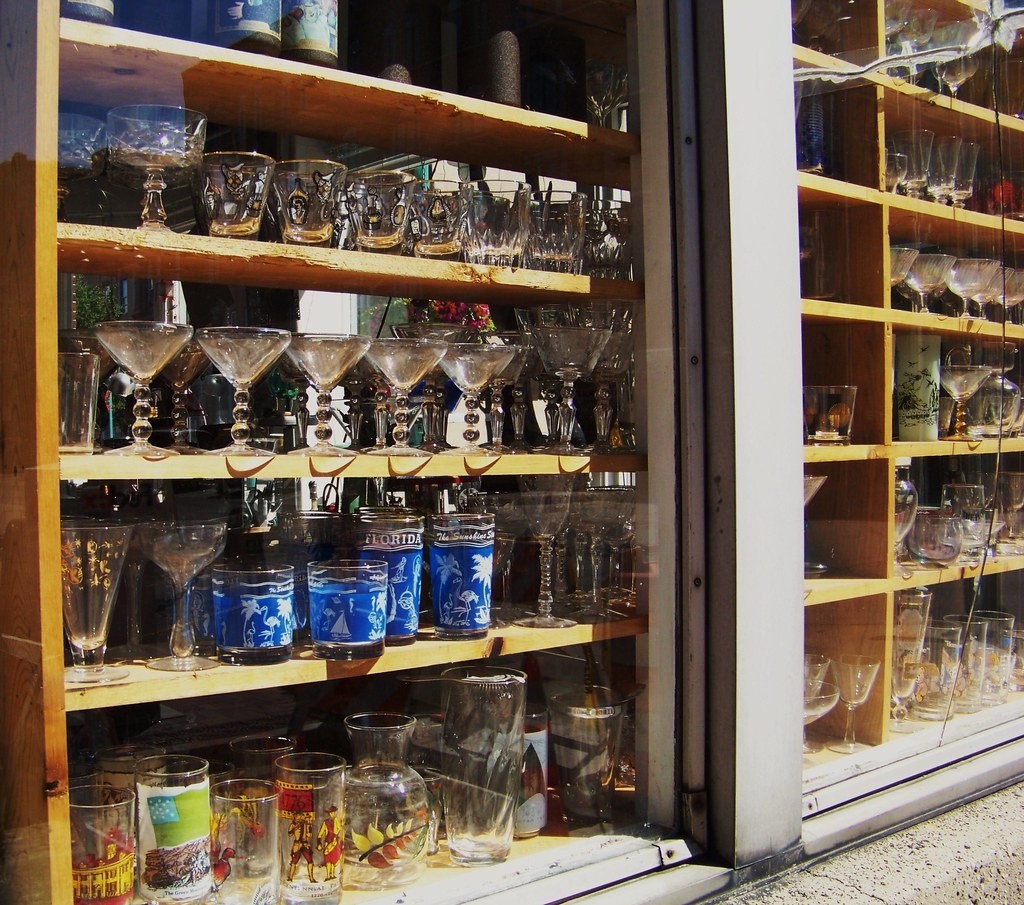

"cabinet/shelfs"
[0,0,709,905]
[791,46,1024,821]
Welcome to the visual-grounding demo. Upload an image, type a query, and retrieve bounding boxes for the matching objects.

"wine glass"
[941,365,992,441]
[891,590,931,732]
[107,104,207,233]
[890,248,1024,324]
[61,474,636,684]
[803,653,881,754]
[885,130,980,210]
[585,57,628,127]
[999,472,1024,536]
[58,299,635,456]
[791,0,979,98]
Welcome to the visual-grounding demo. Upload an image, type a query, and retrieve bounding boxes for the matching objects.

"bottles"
[344,712,428,890]
[967,341,1021,439]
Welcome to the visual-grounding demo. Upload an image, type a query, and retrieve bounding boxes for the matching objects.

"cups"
[428,513,496,641]
[461,180,531,269]
[938,397,955,438]
[267,160,348,249]
[519,190,588,275]
[938,484,985,565]
[513,704,549,838]
[307,559,387,659]
[346,170,415,255]
[67,744,281,905]
[275,753,346,905]
[357,515,425,646]
[441,665,527,867]
[568,199,632,282]
[212,564,293,666]
[201,151,275,241]
[907,514,963,568]
[230,736,296,781]
[550,687,627,826]
[58,354,100,455]
[914,610,1024,721]
[802,386,857,446]
[409,180,474,261]
